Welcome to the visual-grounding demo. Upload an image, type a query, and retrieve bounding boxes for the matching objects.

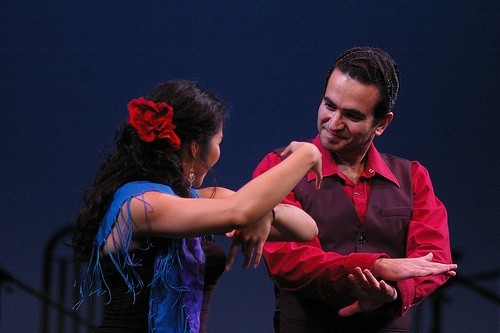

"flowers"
[127,97,182,150]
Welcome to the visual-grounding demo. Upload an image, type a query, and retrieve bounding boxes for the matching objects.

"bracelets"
[272,209,277,224]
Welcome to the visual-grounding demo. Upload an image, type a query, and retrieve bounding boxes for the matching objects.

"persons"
[71,80,324,333]
[251,45,457,333]
